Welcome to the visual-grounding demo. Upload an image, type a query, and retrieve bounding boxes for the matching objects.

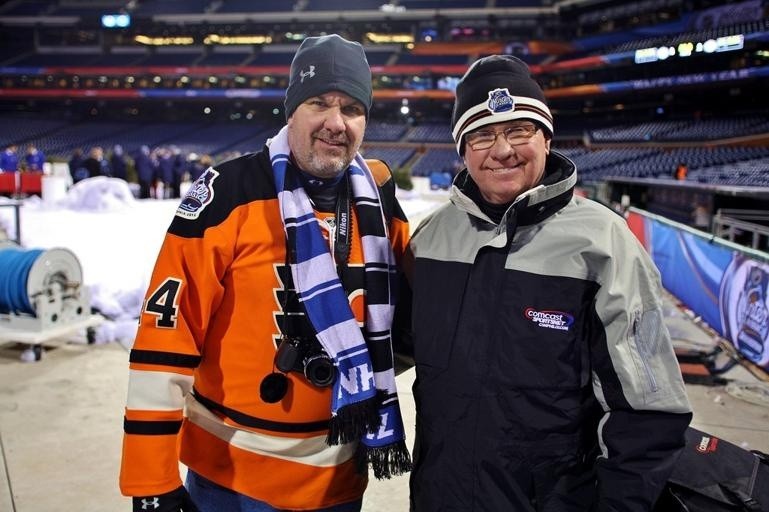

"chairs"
[1,109,286,200]
[553,110,768,188]
[606,21,768,52]
[357,108,466,175]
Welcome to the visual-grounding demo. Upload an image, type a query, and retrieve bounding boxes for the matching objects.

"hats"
[450,54,554,156]
[284,33,373,130]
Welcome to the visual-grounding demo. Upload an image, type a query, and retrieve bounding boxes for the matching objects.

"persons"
[391,55,693,512]
[118,34,410,512]
[0,139,241,200]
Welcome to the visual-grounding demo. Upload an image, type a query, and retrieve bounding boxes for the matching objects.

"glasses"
[462,123,542,151]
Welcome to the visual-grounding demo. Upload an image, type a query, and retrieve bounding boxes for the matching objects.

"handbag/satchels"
[660,425,768,510]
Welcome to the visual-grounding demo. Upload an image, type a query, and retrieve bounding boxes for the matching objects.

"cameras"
[272,337,336,390]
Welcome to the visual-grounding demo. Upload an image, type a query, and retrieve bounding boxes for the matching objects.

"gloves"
[133,483,201,512]
